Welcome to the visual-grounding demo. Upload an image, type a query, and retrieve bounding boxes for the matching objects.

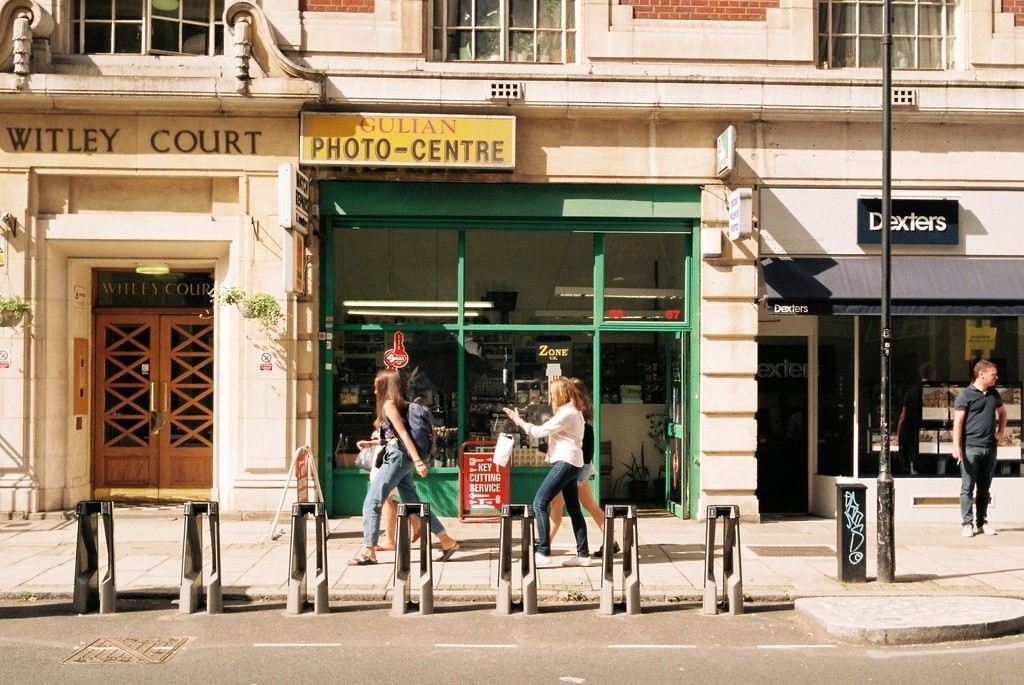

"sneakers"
[978,523,997,535]
[562,555,592,567]
[533,552,552,566]
[963,525,974,537]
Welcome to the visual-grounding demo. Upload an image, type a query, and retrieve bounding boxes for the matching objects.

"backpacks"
[381,401,436,461]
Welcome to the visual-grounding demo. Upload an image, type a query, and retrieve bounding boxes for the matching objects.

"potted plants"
[0,293,34,326]
[610,438,650,501]
[198,285,287,344]
[644,409,671,502]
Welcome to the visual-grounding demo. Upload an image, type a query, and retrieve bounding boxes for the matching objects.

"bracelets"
[413,459,425,467]
[502,375,621,569]
[516,418,522,427]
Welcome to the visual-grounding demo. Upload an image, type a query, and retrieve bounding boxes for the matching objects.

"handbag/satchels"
[354,445,374,471]
[491,411,515,466]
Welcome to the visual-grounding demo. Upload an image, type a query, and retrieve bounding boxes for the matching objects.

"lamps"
[136,266,169,274]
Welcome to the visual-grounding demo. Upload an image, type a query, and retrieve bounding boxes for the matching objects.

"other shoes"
[592,541,620,556]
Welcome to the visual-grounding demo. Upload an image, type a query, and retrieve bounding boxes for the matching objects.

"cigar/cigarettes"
[957,458,961,465]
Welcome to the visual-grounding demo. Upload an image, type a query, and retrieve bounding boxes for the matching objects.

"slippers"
[346,554,378,566]
[436,540,460,561]
[376,543,385,552]
[412,531,421,543]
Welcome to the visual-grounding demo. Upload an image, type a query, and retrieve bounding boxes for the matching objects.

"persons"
[952,359,1007,539]
[346,365,460,567]
[897,361,940,475]
